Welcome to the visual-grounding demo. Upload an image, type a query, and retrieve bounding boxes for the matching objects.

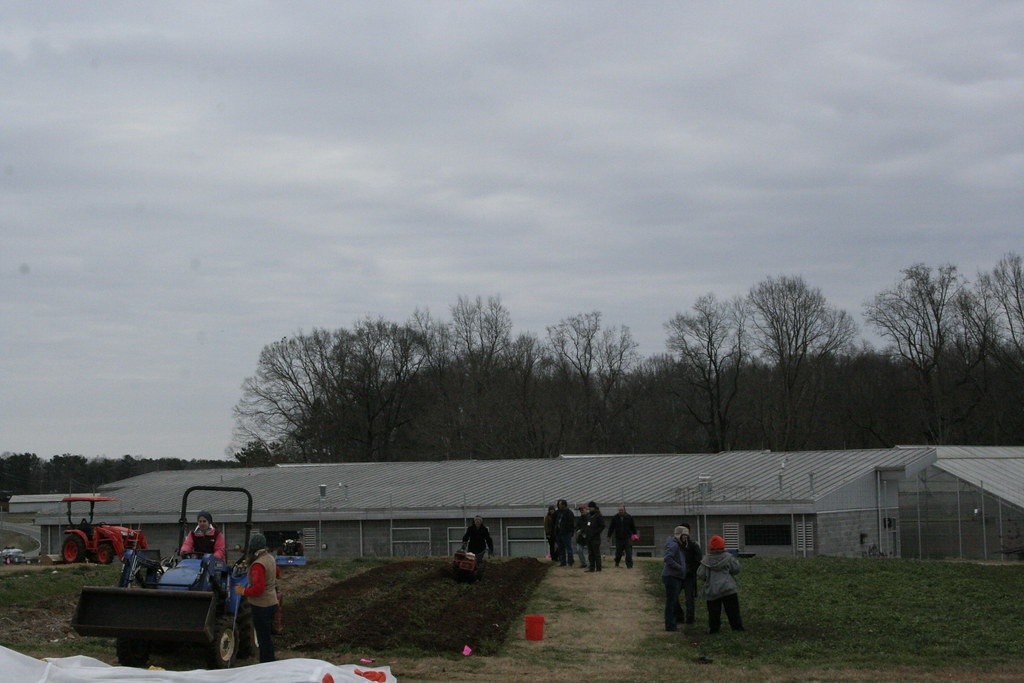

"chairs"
[80,518,91,534]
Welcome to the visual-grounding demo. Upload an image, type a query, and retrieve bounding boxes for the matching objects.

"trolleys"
[452,541,495,584]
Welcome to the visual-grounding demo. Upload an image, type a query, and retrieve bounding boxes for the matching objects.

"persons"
[607,505,637,568]
[697,535,745,634]
[584,502,605,573]
[180,511,225,561]
[462,515,493,558]
[552,500,575,567]
[234,533,280,663]
[661,525,690,630]
[575,505,588,568]
[681,522,701,623]
[544,506,559,560]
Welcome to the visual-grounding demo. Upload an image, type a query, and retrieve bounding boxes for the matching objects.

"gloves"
[235,586,245,595]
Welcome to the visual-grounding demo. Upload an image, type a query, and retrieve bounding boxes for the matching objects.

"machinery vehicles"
[275,554,308,568]
[60,473,148,565]
[69,486,284,670]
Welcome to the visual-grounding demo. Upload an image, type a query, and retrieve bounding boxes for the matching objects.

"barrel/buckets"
[525,615,544,640]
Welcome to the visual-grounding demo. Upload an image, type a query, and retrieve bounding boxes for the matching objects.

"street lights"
[317,482,327,559]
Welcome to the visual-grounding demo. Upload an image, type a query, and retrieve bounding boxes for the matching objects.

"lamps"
[322,543,328,550]
[860,533,867,544]
[234,544,242,549]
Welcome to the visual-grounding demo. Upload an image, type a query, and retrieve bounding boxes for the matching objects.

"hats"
[709,536,724,550]
[247,533,266,552]
[579,507,587,510]
[673,527,690,548]
[197,511,212,524]
[474,515,483,524]
